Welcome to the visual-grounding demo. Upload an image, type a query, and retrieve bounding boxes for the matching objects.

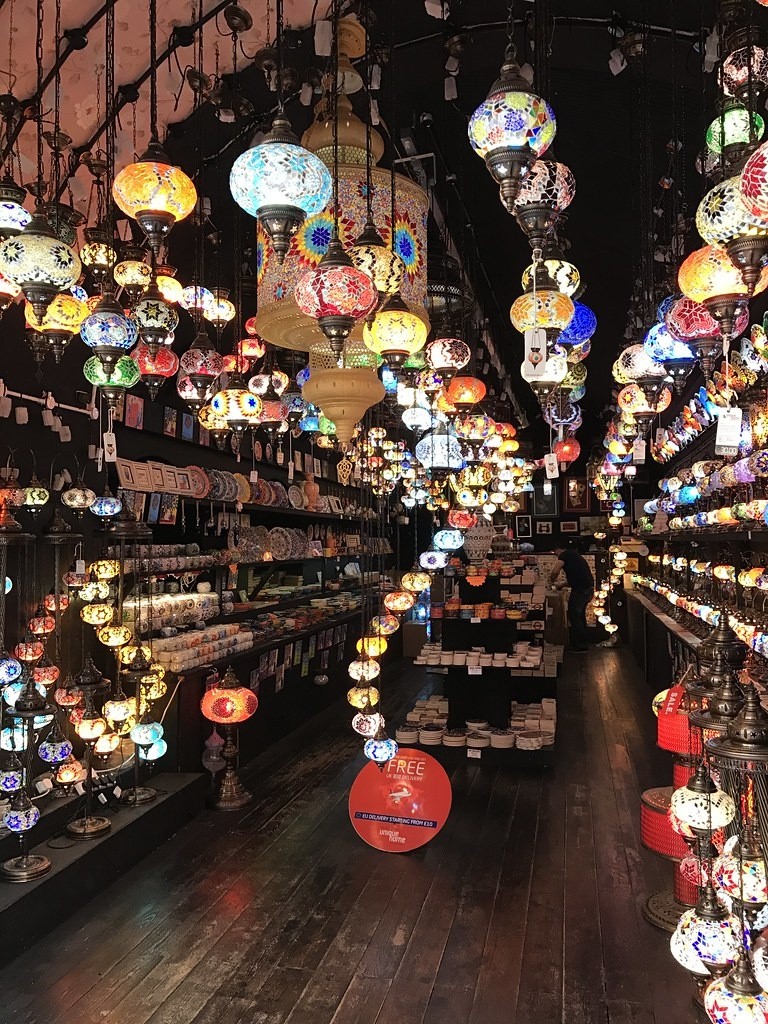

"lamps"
[671,606,768,1024]
[201,666,257,810]
[0,653,156,883]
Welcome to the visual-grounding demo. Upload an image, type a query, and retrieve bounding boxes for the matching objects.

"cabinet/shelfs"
[610,288,768,715]
[102,424,402,789]
[396,576,558,774]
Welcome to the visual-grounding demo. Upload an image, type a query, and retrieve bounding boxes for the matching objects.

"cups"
[144,621,254,672]
[222,590,235,616]
[120,581,220,633]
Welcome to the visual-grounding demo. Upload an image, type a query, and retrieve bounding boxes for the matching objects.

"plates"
[288,485,305,509]
[320,525,325,540]
[228,524,308,563]
[184,465,288,508]
[327,526,332,539]
[307,523,314,542]
[314,524,319,539]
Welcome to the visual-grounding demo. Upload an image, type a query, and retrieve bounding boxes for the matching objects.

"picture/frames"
[329,496,338,513]
[506,492,527,513]
[516,516,533,538]
[346,534,360,547]
[634,499,653,521]
[533,484,560,519]
[564,477,591,513]
[116,458,139,495]
[148,460,169,493]
[177,468,195,496]
[134,462,153,492]
[334,496,344,515]
[165,465,181,494]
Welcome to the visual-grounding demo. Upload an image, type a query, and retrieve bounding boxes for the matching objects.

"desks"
[548,589,568,646]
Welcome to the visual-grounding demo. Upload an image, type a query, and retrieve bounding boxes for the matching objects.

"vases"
[490,525,510,560]
[463,512,494,565]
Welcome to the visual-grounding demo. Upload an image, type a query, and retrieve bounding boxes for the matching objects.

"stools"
[403,620,431,658]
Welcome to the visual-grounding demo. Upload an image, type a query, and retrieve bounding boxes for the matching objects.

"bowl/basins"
[310,591,367,618]
[250,584,322,601]
[330,584,339,590]
[324,576,359,587]
